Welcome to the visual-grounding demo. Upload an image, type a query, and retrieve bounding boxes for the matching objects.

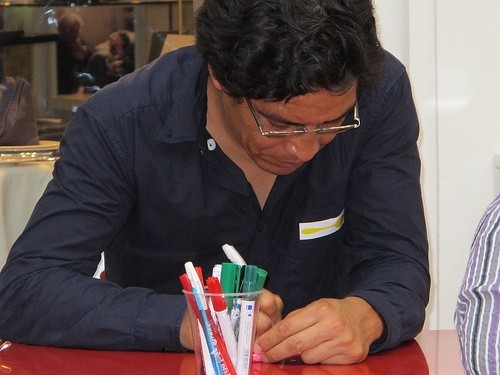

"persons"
[0,0,432,365]
[57,7,134,96]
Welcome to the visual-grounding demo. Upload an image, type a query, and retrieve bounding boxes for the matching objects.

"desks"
[0,339,428,375]
[1,139,110,284]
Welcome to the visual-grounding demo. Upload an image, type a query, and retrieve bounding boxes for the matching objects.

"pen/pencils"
[180,243,268,375]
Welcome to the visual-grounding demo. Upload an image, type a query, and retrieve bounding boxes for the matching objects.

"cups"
[182,286,264,375]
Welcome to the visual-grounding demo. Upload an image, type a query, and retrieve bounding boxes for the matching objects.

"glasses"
[243,94,361,138]
